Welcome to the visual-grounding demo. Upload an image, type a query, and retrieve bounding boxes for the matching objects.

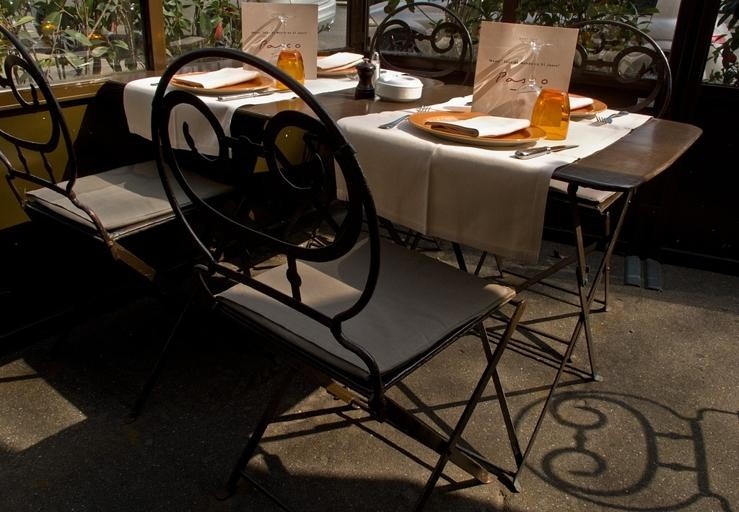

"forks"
[593,109,630,124]
[377,104,431,130]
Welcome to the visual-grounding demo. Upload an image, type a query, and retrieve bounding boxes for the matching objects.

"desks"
[103,56,706,493]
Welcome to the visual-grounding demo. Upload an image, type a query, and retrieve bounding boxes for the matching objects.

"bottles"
[353,58,377,101]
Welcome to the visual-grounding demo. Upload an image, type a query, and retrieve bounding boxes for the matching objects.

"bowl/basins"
[374,73,424,102]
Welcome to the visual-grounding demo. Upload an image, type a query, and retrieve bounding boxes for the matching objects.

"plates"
[566,90,608,119]
[407,110,547,149]
[316,55,366,76]
[170,66,274,96]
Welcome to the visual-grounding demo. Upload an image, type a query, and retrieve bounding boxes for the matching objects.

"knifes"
[514,144,581,159]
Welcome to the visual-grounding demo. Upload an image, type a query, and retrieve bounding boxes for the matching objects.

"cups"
[531,87,572,140]
[274,49,305,91]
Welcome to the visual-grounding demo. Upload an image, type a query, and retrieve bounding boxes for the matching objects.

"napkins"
[426,116,530,138]
[174,68,261,90]
[317,52,364,72]
[569,97,594,110]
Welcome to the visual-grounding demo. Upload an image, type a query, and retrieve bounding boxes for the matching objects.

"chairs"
[473,19,673,334]
[150,46,523,512]
[371,2,475,87]
[0,25,257,424]
